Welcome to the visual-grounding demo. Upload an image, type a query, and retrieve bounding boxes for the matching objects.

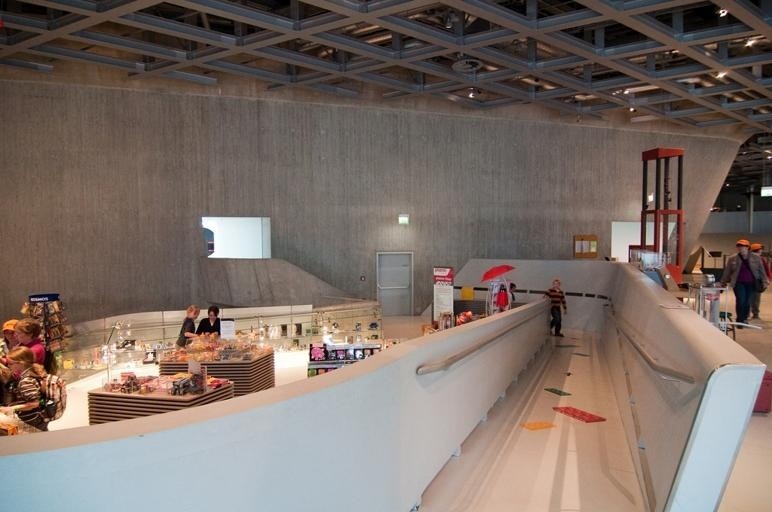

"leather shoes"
[555,331,564,337]
[737,317,744,329]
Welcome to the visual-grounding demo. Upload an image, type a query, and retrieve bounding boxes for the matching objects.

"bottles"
[111,379,121,391]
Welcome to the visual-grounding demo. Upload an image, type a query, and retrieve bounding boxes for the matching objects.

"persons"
[751,243,772,318]
[509,282,516,302]
[175,304,199,354]
[196,305,221,342]
[1,317,67,431]
[721,239,770,329]
[544,279,568,337]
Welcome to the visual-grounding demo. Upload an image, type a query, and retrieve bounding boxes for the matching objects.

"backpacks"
[17,373,67,419]
[45,350,58,373]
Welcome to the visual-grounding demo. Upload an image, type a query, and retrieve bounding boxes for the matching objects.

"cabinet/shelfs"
[307,341,381,376]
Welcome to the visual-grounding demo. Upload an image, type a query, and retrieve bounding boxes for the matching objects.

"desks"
[88,346,276,426]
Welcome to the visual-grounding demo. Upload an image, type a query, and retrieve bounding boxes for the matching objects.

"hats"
[736,239,762,251]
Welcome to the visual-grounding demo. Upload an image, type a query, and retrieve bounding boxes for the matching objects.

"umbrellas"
[481,264,515,282]
